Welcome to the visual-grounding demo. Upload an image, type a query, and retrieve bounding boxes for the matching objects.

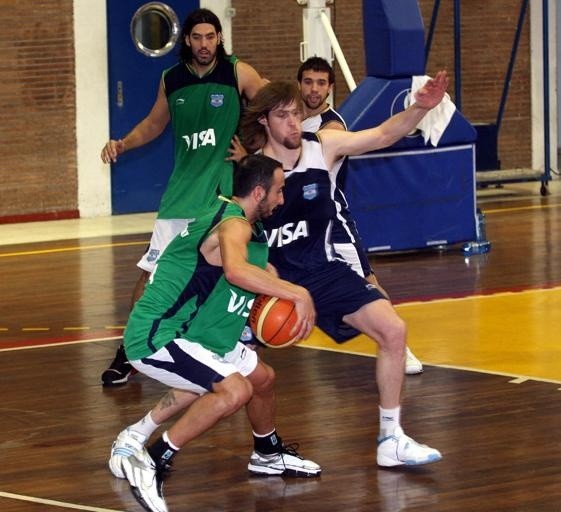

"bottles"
[459,241,490,256]
[476,208,487,241]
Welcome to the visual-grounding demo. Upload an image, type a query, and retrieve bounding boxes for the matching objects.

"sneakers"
[100,345,139,386]
[376,428,443,471]
[121,445,170,512]
[405,345,424,377]
[248,448,323,478]
[109,424,146,480]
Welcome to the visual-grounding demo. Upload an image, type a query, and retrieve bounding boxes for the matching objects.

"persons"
[107,69,450,479]
[120,153,322,512]
[224,57,423,374]
[101,8,268,386]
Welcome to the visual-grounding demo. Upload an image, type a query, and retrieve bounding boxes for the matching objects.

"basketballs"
[249,292,306,349]
